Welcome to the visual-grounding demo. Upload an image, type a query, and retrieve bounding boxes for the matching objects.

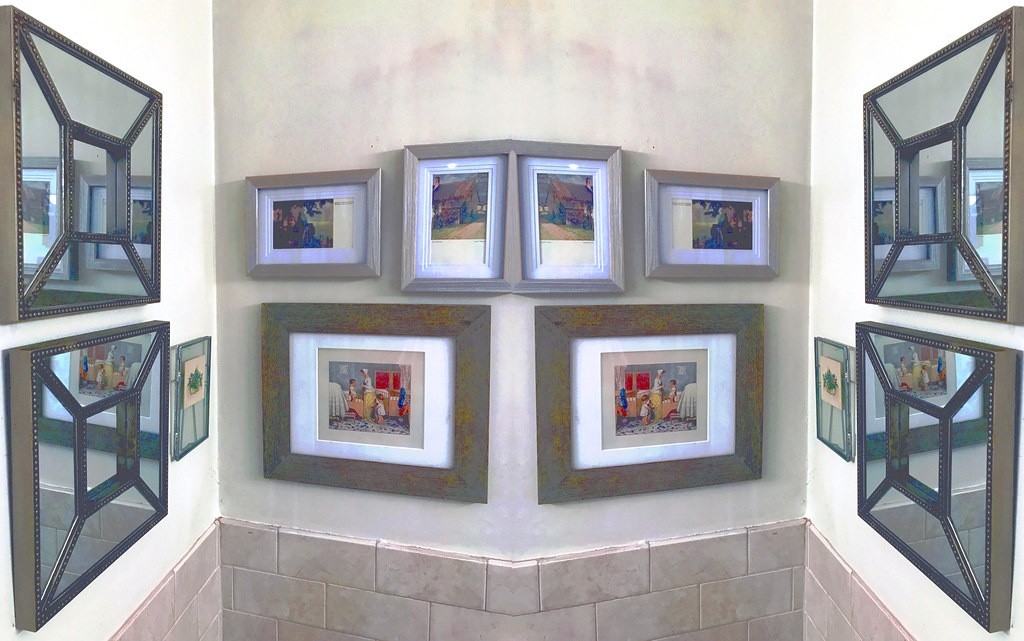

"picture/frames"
[950,152,1006,284]
[510,136,625,293]
[78,175,153,271]
[644,165,782,280]
[68,334,149,414]
[246,168,383,280]
[401,141,510,293]
[536,302,763,507]
[813,336,853,461]
[175,336,209,461]
[20,155,76,281]
[262,302,492,504]
[872,334,959,418]
[873,174,943,272]
